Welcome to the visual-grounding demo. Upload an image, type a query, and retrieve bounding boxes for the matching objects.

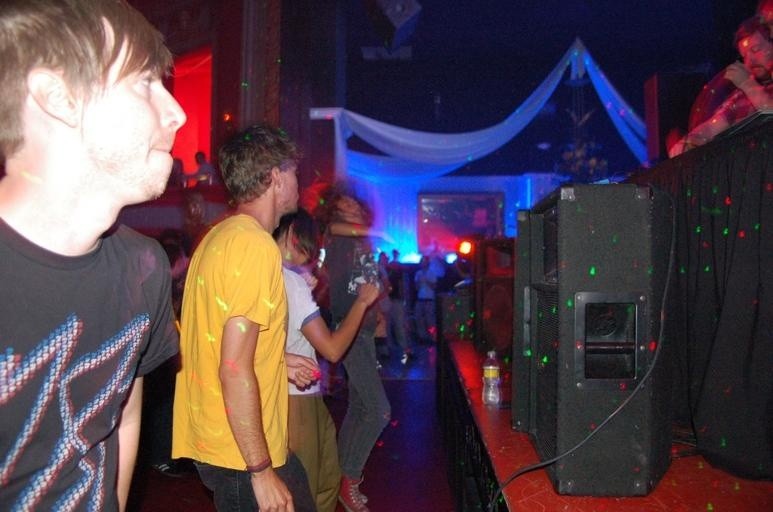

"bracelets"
[245,459,273,476]
[351,223,362,237]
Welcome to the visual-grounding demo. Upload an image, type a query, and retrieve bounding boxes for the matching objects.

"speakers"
[437,295,477,440]
[530,184,676,499]
[477,242,515,357]
[512,210,545,431]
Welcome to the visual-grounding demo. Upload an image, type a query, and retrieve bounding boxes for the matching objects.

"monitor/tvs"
[416,190,504,254]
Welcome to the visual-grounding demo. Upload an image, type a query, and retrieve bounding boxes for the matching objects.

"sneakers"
[337,479,369,512]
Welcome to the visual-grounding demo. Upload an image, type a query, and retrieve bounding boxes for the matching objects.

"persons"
[374,253,392,369]
[172,123,321,511]
[725,17,772,127]
[324,194,392,512]
[1,1,188,511]
[272,206,380,510]
[414,255,437,345]
[143,242,190,479]
[388,250,408,364]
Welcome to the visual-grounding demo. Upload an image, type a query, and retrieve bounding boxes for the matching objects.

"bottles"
[481,354,501,409]
